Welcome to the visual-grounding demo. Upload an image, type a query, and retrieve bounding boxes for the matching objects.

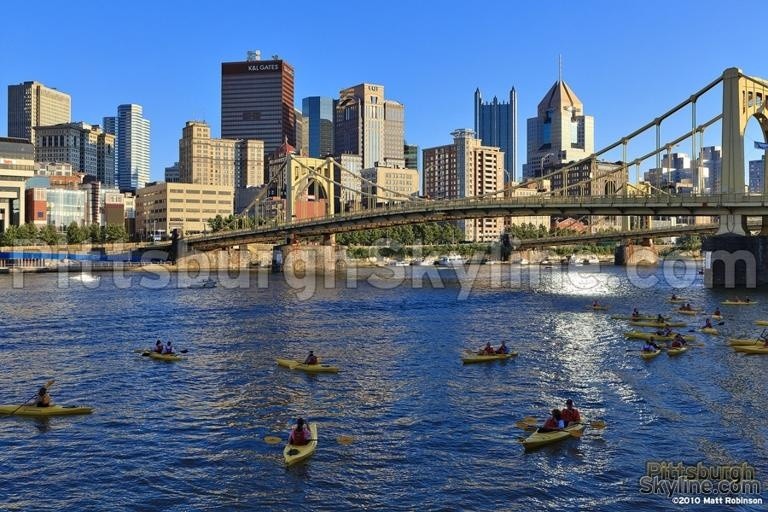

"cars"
[152,235,162,241]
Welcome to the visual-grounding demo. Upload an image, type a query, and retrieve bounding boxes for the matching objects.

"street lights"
[540,152,555,190]
[498,168,511,199]
[153,219,158,245]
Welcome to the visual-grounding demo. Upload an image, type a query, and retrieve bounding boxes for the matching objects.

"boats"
[522,420,587,449]
[1,402,95,416]
[147,351,189,361]
[458,349,517,363]
[586,294,767,358]
[277,356,341,375]
[282,421,320,468]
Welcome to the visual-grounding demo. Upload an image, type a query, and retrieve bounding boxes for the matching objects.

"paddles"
[306,436,352,444]
[11,380,55,415]
[163,349,188,355]
[524,417,604,428]
[682,344,705,347]
[713,322,725,328]
[265,436,289,443]
[466,350,508,359]
[625,349,650,352]
[518,422,582,438]
[135,349,156,353]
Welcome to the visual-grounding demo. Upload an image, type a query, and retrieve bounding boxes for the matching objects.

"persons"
[543,399,580,431]
[37,387,50,407]
[288,418,311,445]
[154,340,176,354]
[485,340,509,354]
[632,294,721,353]
[305,351,317,365]
[733,296,750,302]
[758,330,768,348]
[592,299,600,307]
